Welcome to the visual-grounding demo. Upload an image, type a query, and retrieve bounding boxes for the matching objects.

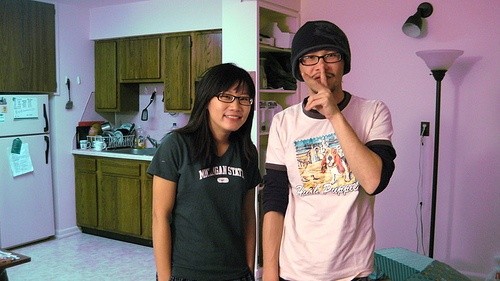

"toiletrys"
[138,136,145,149]
[260,58,267,88]
[134,127,144,148]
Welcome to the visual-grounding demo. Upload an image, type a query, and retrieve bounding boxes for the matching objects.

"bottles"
[137,136,145,150]
[171,123,179,131]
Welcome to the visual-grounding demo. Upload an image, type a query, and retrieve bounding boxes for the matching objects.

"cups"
[80,140,92,150]
[94,142,107,152]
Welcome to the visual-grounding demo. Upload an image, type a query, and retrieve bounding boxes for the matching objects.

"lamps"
[415,49,465,259]
[402,2,433,37]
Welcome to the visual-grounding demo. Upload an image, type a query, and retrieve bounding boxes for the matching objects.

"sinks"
[94,148,156,156]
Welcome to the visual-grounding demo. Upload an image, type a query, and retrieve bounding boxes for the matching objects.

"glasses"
[298,52,345,66]
[215,92,254,106]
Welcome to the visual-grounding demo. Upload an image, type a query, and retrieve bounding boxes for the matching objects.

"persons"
[146,63,260,281]
[260,21,396,281]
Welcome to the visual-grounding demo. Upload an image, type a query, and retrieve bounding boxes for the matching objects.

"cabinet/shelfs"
[73,155,153,248]
[258,0,302,191]
[93,28,222,113]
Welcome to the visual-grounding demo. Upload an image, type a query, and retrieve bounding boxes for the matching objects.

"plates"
[103,130,124,148]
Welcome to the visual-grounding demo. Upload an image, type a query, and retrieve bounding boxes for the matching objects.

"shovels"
[141,91,156,121]
[66,78,73,110]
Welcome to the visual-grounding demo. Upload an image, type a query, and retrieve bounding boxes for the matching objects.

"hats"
[291,20,351,82]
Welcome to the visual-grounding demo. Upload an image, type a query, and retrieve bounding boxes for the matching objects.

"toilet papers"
[289,34,295,48]
[273,23,289,48]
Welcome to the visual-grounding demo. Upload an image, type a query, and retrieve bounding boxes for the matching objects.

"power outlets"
[420,122,430,136]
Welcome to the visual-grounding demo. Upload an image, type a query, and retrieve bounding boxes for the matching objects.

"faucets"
[145,135,160,148]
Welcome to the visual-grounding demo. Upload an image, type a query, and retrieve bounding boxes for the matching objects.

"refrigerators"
[0,93,56,248]
